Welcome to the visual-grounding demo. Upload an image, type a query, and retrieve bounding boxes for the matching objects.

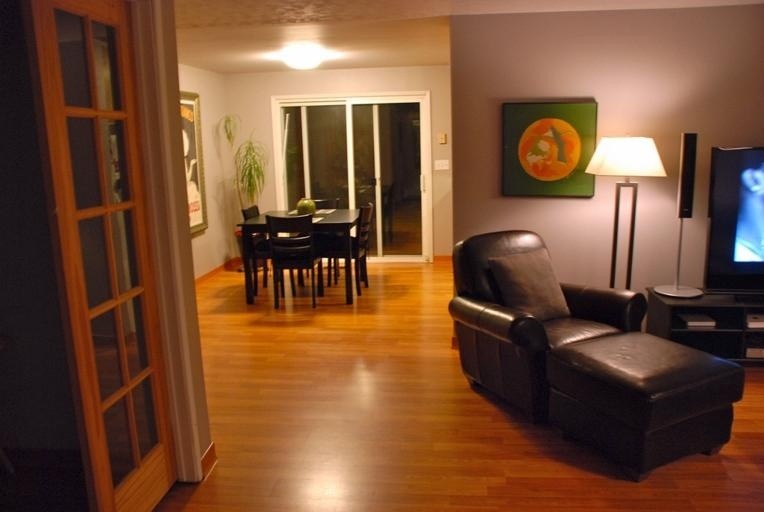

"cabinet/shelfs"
[643,279,763,364]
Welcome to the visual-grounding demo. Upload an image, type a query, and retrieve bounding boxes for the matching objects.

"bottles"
[296,197,315,216]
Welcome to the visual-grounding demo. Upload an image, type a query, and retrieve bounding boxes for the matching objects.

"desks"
[236,209,360,306]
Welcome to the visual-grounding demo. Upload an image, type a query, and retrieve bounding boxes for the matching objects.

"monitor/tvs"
[703,145,764,291]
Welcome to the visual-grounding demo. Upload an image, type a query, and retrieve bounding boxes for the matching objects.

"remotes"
[719,145,753,151]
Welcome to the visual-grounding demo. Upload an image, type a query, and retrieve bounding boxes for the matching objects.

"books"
[679,312,716,327]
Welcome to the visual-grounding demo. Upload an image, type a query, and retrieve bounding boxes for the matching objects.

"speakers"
[676,131,698,219]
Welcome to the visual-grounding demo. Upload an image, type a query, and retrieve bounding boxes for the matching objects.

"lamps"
[584,132,669,291]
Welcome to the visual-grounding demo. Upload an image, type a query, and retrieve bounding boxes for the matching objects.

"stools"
[542,327,748,484]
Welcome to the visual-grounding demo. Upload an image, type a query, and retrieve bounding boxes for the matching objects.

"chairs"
[446,225,651,428]
[264,213,320,310]
[240,204,273,286]
[302,197,340,288]
[320,201,373,295]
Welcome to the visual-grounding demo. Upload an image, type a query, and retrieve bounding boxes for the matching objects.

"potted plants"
[218,108,276,270]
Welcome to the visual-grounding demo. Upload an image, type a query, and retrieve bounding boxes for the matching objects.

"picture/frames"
[500,96,599,199]
[180,88,208,235]
